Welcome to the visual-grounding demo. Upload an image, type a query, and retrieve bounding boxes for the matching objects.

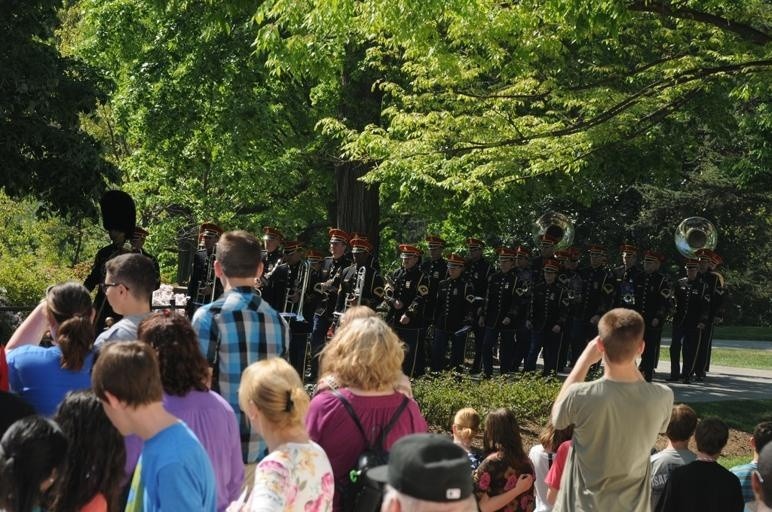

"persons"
[528,417,574,511]
[226,357,335,510]
[191,229,289,502]
[551,308,674,511]
[260,225,724,383]
[93,341,218,512]
[186,223,223,320]
[366,434,474,512]
[453,407,532,511]
[473,408,536,512]
[84,190,161,329]
[313,306,413,398]
[0,349,128,510]
[5,283,101,416]
[125,309,244,510]
[650,403,770,511]
[306,316,426,511]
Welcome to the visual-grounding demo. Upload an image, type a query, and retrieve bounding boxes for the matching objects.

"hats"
[261,227,374,262]
[130,227,149,237]
[366,432,472,501]
[101,190,135,238]
[201,224,223,238]
[399,236,721,273]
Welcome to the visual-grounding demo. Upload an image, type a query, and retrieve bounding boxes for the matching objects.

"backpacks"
[333,390,409,512]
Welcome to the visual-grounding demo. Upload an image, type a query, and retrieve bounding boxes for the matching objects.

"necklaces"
[696,457,715,460]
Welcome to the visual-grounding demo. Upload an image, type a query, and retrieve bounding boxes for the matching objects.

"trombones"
[283,259,310,324]
[343,265,367,310]
[192,246,217,311]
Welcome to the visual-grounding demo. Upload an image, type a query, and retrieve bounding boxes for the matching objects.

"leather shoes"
[665,374,704,384]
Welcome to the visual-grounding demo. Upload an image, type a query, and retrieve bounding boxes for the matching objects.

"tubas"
[674,216,719,260]
[533,212,575,256]
